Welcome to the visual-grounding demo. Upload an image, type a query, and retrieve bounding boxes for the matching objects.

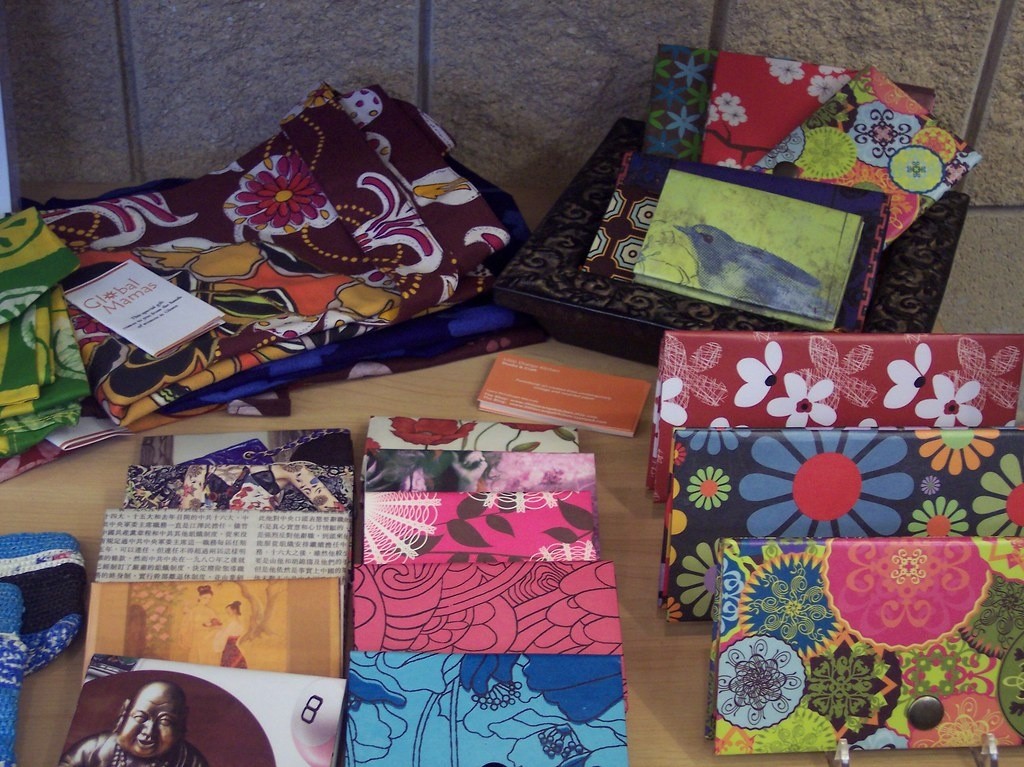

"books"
[477,352,651,438]
